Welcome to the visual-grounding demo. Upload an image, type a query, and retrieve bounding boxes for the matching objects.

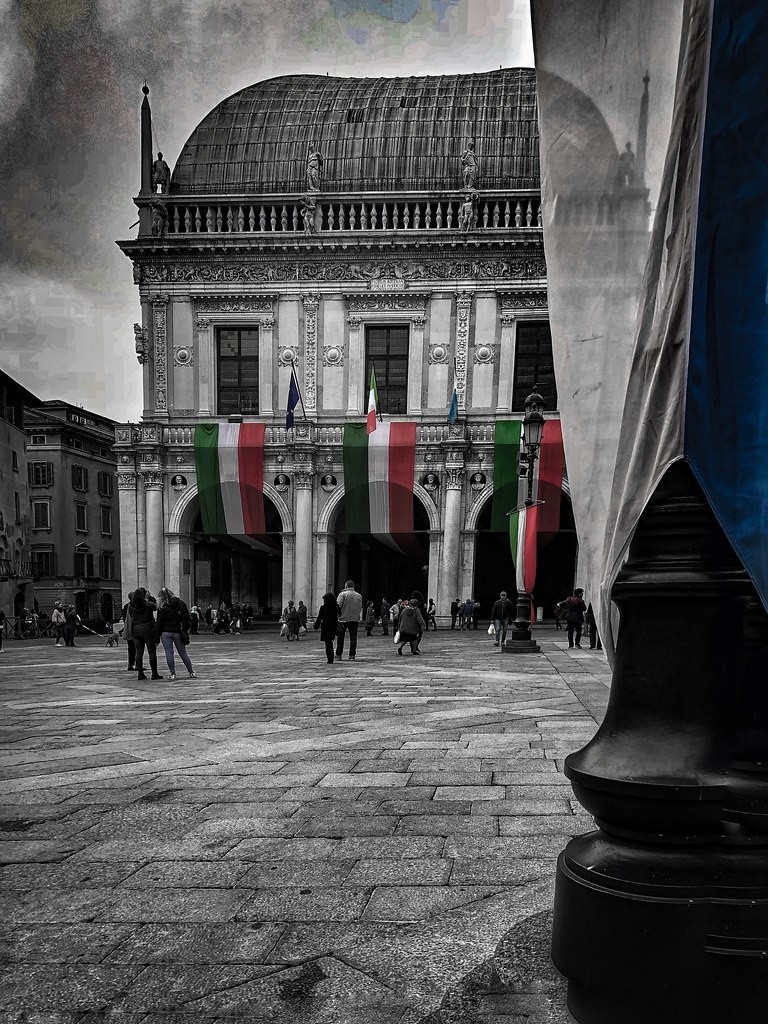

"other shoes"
[151,674,164,680]
[412,651,420,655]
[334,655,342,661]
[568,644,583,650]
[135,667,147,671]
[127,667,135,671]
[188,672,197,678]
[138,674,147,680]
[494,640,505,648]
[398,648,402,655]
[348,656,355,661]
[168,674,177,680]
[55,643,63,647]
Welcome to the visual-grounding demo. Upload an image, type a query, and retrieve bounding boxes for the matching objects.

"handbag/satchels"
[336,623,345,636]
[280,623,289,637]
[179,629,191,646]
[299,625,307,637]
[123,604,133,641]
[394,631,401,645]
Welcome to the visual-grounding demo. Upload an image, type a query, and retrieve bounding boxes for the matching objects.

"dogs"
[105,633,119,647]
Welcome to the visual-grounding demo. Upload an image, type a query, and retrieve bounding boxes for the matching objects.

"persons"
[155,587,196,679]
[276,475,289,491]
[491,588,602,652]
[172,476,187,490]
[424,475,437,491]
[122,592,146,670]
[128,587,163,679]
[0,602,81,651]
[279,580,482,665]
[472,474,484,490]
[188,602,254,635]
[322,475,335,491]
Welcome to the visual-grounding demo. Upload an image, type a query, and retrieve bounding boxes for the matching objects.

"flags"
[449,391,457,425]
[286,372,298,431]
[366,365,379,434]
[343,422,417,533]
[491,420,563,532]
[194,423,266,535]
[509,506,537,626]
